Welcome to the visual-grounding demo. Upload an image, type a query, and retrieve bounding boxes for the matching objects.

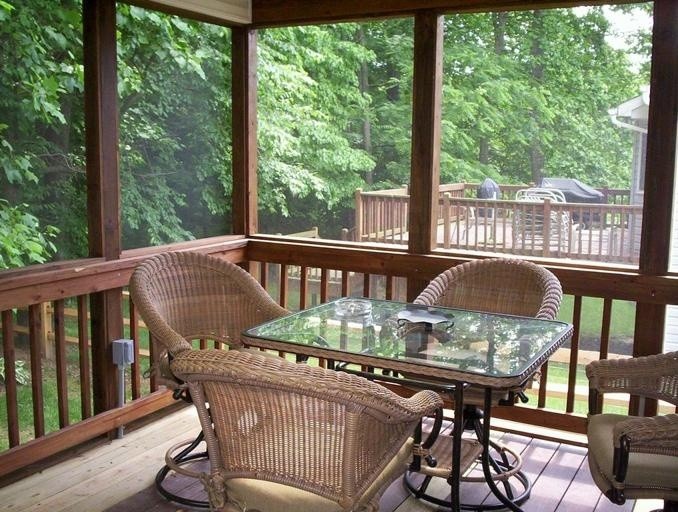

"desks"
[241,295,575,512]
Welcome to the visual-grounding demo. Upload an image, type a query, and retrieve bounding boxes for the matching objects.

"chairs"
[584,350,678,511]
[515,186,577,254]
